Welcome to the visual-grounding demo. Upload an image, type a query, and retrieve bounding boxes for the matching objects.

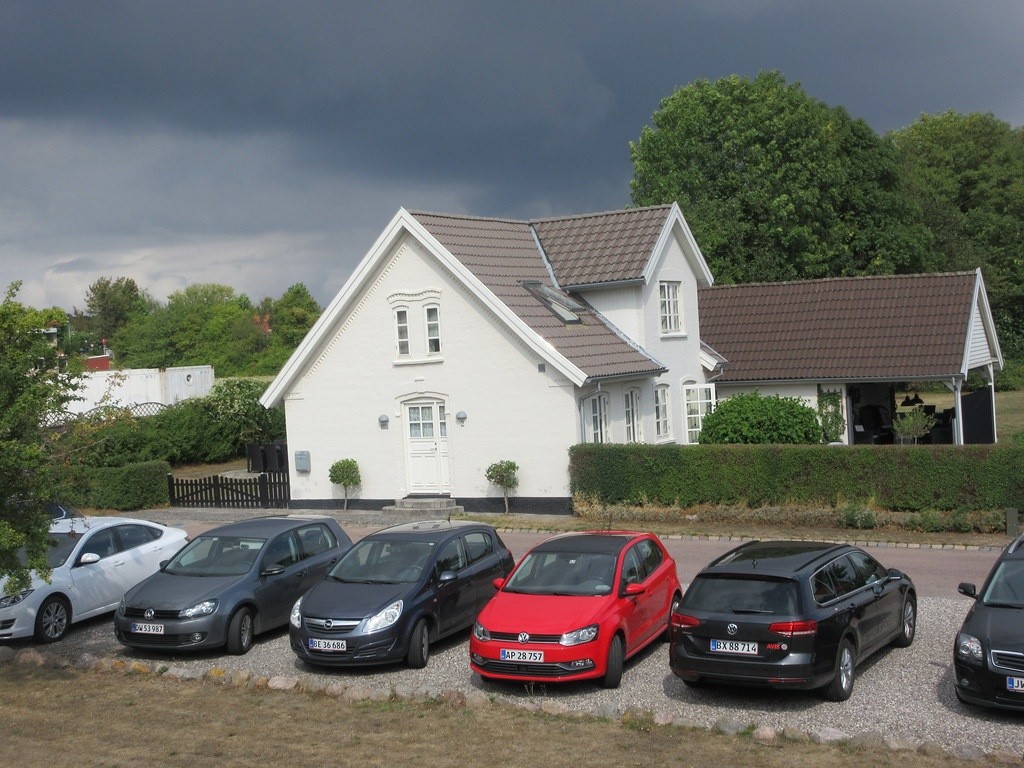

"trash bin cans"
[872,425,893,445]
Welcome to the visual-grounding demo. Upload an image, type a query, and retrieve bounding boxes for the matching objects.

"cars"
[113,512,357,656]
[0,517,194,644]
[0,491,84,522]
[288,520,518,671]
[468,528,685,689]
[950,534,1024,714]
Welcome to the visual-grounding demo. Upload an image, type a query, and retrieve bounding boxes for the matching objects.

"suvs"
[667,537,919,703]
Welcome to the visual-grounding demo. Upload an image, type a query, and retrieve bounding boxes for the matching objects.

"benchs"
[297,529,329,554]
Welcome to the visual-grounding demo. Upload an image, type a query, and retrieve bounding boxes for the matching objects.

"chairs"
[851,405,955,445]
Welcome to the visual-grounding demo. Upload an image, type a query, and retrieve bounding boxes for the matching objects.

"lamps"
[899,382,924,407]
[456,411,467,427]
[378,415,389,429]
